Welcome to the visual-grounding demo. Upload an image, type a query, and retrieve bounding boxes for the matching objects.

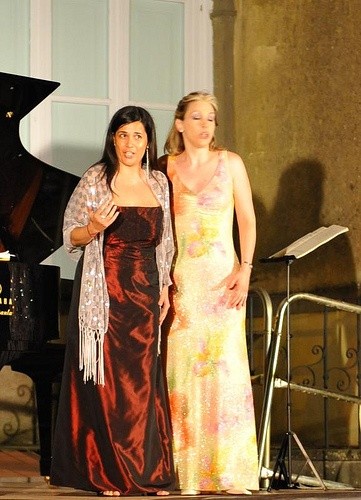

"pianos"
[0,70,83,491]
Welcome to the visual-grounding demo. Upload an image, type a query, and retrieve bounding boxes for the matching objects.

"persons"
[153,90,260,495]
[50,105,175,497]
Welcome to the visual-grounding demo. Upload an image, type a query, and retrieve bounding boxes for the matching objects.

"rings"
[101,215,105,218]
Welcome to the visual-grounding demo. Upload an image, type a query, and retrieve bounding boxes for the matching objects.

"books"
[267,225,349,259]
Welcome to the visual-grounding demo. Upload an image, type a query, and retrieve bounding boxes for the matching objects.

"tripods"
[259,224,350,491]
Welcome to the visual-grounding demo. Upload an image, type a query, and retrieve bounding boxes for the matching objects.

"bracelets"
[87,225,93,238]
[242,261,253,269]
